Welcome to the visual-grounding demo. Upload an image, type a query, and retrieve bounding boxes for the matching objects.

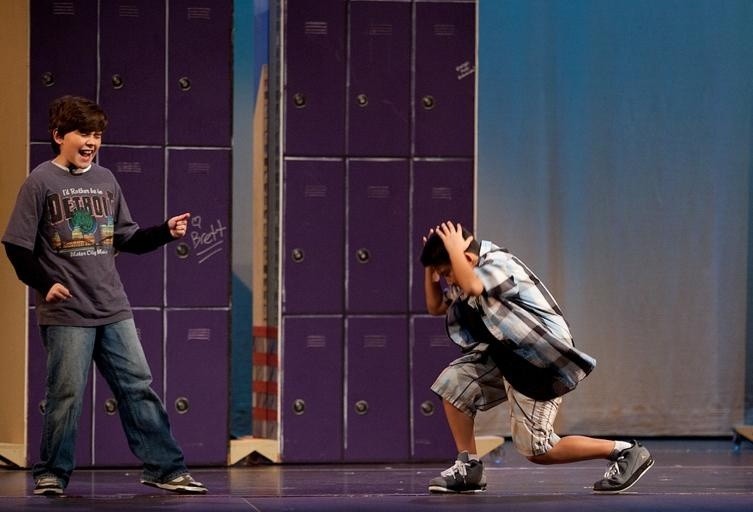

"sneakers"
[592,439,656,494]
[139,470,209,494]
[428,450,488,494]
[32,472,63,495]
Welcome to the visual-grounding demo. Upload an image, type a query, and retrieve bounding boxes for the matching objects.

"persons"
[420,221,656,494]
[1,95,211,495]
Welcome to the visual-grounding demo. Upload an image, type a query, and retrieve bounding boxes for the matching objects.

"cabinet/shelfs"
[253,1,481,466]
[0,1,236,472]
